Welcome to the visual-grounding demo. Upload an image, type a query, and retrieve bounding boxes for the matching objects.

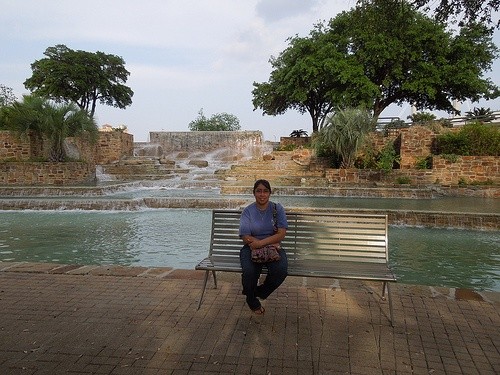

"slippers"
[253,306,265,315]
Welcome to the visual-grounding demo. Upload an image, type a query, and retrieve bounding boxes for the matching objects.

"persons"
[239,180,288,314]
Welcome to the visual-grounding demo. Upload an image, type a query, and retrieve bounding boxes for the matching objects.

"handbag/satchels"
[250,243,282,263]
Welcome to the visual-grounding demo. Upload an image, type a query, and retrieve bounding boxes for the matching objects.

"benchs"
[195,210,398,325]
